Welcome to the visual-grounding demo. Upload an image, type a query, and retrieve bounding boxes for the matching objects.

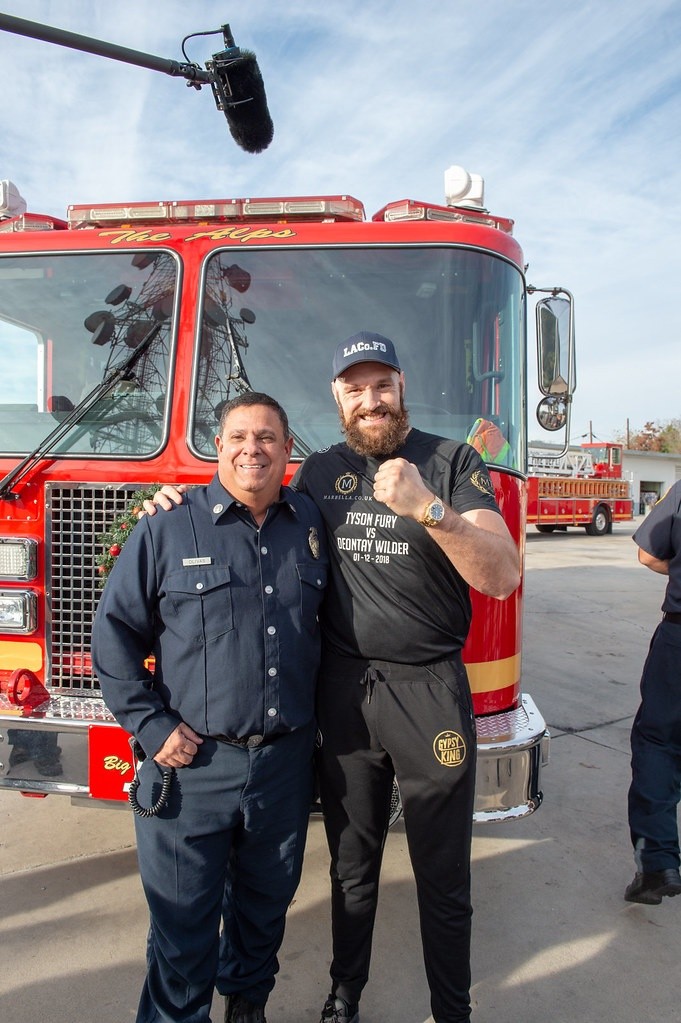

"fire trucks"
[0,165,579,826]
[525,439,636,537]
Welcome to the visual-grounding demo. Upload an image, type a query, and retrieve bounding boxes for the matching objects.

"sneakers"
[625,868,681,905]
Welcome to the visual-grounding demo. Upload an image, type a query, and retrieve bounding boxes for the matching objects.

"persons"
[90,331,524,1023]
[624,478,681,905]
[540,411,565,429]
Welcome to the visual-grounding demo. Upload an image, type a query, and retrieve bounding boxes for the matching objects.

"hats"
[333,331,401,383]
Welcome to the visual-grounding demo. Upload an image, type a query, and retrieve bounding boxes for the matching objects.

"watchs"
[417,495,445,527]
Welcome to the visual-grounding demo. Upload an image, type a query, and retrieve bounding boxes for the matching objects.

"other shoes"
[33,751,63,777]
[224,994,267,1023]
[8,743,30,766]
[317,994,359,1023]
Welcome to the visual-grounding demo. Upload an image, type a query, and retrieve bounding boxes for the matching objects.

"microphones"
[211,24,274,153]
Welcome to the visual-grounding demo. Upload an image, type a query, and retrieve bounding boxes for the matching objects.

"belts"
[665,611,681,623]
